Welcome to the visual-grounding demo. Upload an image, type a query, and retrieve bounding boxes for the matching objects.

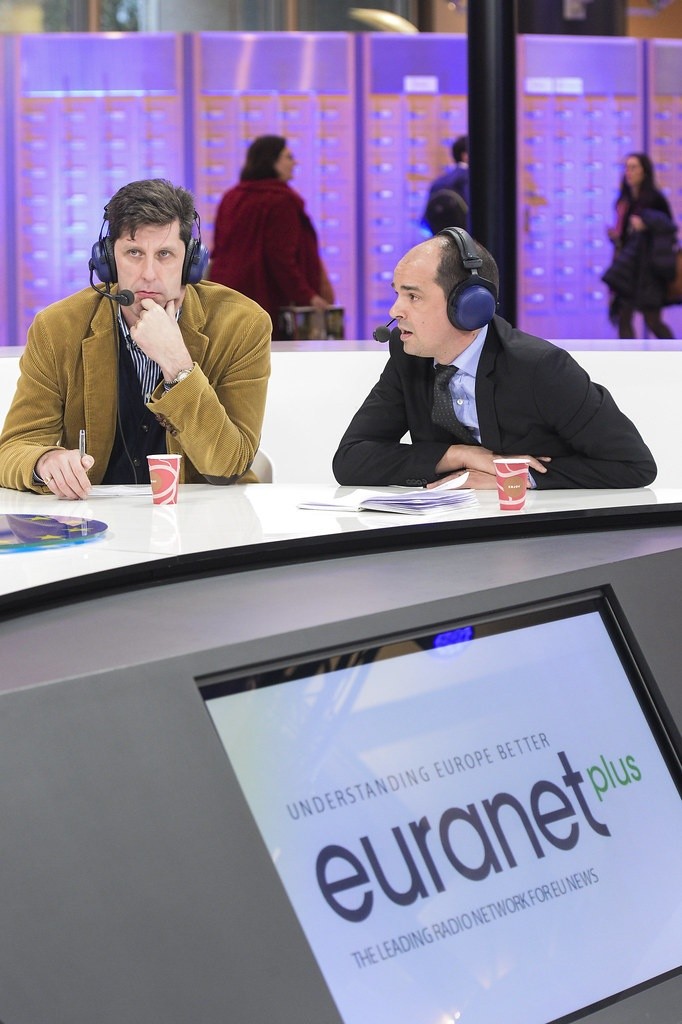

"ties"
[431,363,480,446]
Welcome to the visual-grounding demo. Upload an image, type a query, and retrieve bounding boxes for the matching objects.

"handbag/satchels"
[665,248,682,307]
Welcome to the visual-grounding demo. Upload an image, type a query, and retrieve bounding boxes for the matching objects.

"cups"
[493,458,531,511]
[146,454,182,507]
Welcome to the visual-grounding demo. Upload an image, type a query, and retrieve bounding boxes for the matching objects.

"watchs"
[164,367,194,389]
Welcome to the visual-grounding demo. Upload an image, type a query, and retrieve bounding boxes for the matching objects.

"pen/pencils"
[79,429,85,458]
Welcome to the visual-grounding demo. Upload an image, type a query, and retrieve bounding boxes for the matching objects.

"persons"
[417,133,470,235]
[205,135,336,342]
[332,236,658,489]
[599,154,677,339]
[0,177,273,500]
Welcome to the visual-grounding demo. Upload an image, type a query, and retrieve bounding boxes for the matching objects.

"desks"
[0,482,682,1024]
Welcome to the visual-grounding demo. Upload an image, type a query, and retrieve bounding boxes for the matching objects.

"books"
[297,473,478,516]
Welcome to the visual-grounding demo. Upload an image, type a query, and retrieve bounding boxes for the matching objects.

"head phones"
[437,225,499,331]
[90,195,207,287]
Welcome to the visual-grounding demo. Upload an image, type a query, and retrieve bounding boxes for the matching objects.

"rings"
[43,474,53,485]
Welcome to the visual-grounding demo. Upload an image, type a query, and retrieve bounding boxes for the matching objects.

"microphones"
[88,267,134,306]
[372,317,396,345]
